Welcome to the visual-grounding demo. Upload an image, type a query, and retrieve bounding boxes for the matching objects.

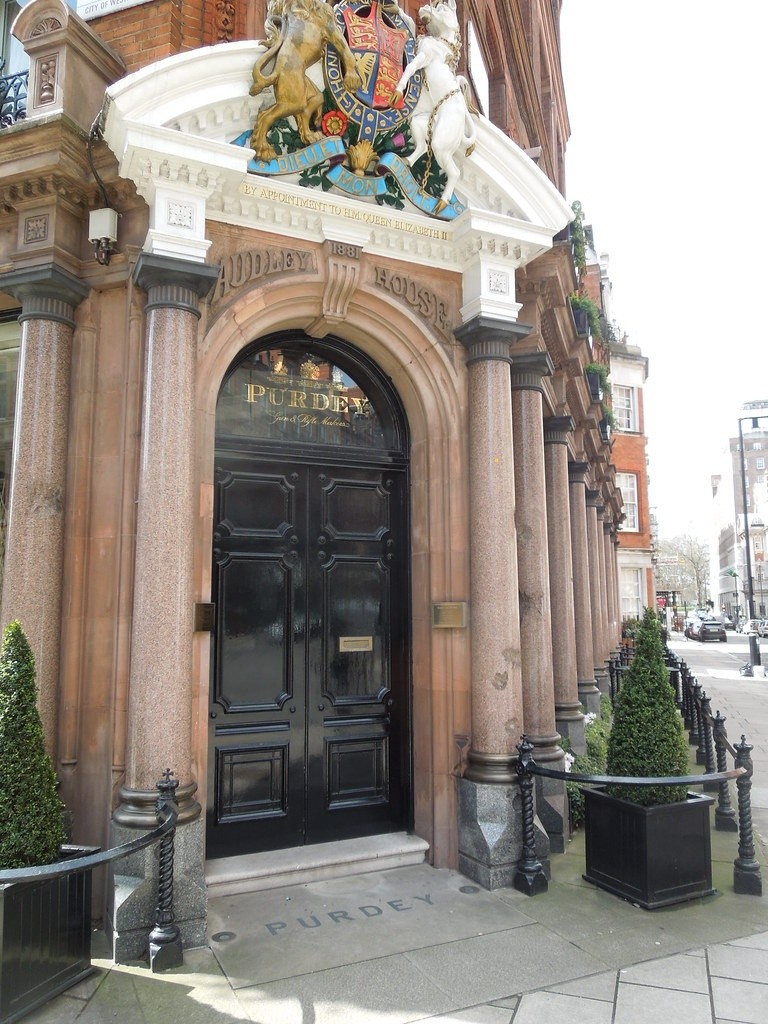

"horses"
[389,0,482,215]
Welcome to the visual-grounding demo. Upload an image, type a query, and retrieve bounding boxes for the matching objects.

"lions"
[247,0,363,167]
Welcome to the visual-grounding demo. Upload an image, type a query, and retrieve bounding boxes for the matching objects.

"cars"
[724,617,735,628]
[735,618,768,637]
[685,607,716,638]
[697,621,727,642]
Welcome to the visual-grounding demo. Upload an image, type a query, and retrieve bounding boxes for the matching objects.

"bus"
[656,590,684,620]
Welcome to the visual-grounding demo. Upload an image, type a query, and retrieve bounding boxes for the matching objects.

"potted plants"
[578,609,717,910]
[0,620,102,1024]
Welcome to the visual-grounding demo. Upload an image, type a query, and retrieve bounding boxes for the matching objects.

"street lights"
[727,569,749,618]
[758,564,765,616]
[738,417,768,617]
